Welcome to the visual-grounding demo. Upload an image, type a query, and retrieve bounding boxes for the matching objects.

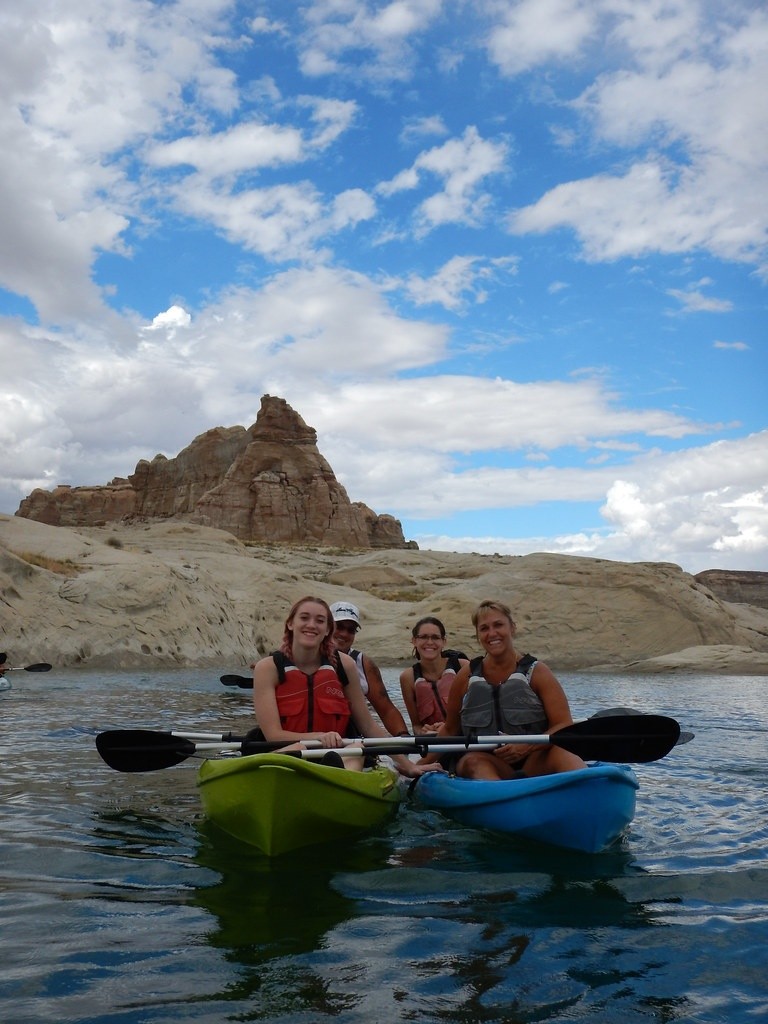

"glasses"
[334,623,359,634]
[415,634,444,641]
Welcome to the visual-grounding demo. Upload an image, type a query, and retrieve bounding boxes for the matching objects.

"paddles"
[93,710,683,775]
[219,674,254,690]
[279,705,648,761]
[4,662,53,673]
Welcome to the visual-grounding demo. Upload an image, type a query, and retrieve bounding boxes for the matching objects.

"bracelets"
[396,731,410,736]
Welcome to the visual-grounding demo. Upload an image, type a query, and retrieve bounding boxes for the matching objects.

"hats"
[328,601,361,629]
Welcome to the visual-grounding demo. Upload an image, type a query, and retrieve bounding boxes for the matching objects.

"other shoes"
[320,751,345,769]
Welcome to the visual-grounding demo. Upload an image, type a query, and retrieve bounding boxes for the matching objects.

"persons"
[400,617,471,736]
[415,600,589,780]
[0,665,5,678]
[253,596,449,778]
[329,601,412,766]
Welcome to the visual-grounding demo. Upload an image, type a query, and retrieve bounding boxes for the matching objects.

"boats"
[411,761,642,857]
[193,753,402,859]
